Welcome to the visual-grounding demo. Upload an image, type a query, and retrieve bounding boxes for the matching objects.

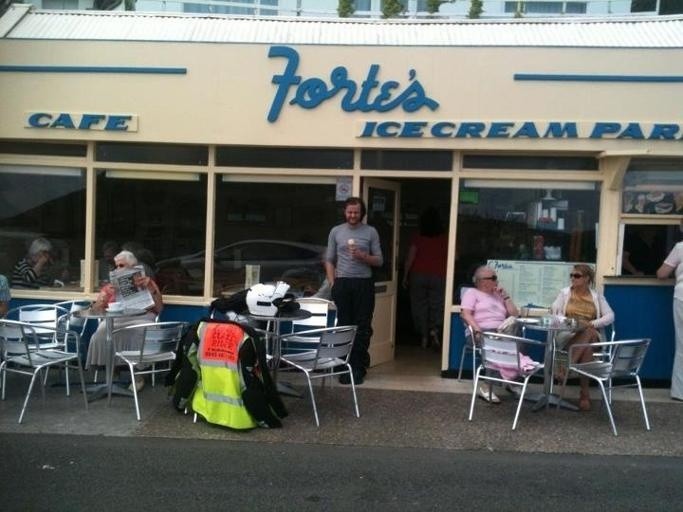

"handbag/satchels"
[503,296,511,304]
[150,289,158,296]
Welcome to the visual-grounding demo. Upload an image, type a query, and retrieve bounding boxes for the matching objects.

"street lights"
[496,316,522,343]
[212,281,300,315]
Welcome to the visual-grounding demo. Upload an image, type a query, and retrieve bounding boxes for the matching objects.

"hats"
[149,235,331,299]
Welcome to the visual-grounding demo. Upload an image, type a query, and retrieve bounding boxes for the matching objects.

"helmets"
[517,318,539,324]
[104,308,122,312]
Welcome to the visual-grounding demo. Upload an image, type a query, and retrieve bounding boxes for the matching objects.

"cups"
[569,272,583,278]
[114,262,128,268]
[481,274,498,281]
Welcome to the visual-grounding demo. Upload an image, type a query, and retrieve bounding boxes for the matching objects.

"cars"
[541,315,553,326]
[107,302,120,310]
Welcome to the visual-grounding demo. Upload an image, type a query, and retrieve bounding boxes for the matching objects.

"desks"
[73,305,147,402]
[245,310,311,397]
[506,311,602,414]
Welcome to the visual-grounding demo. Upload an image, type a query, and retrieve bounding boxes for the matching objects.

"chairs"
[34,298,100,386]
[456,289,526,390]
[279,298,338,389]
[552,315,618,408]
[561,332,657,439]
[106,322,189,421]
[274,325,361,428]
[128,378,144,392]
[3,304,70,396]
[93,313,160,388]
[468,325,556,429]
[1,318,89,424]
[578,390,591,411]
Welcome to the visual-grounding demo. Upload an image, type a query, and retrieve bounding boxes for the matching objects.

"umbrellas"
[501,381,522,395]
[478,386,500,403]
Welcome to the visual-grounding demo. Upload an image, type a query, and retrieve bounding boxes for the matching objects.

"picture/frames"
[506,311,602,414]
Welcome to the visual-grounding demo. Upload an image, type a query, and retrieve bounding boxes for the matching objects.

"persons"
[457,264,527,404]
[655,216,683,401]
[81,251,165,393]
[119,243,161,290]
[400,206,450,353]
[100,242,122,284]
[545,261,619,414]
[8,237,56,289]
[623,225,658,276]
[0,275,13,321]
[318,194,382,386]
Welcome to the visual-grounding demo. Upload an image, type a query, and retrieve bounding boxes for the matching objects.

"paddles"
[0,370,682,461]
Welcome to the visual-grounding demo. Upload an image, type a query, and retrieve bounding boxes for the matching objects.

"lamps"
[456,289,526,390]
[468,325,556,429]
[561,332,657,439]
[552,315,618,408]
